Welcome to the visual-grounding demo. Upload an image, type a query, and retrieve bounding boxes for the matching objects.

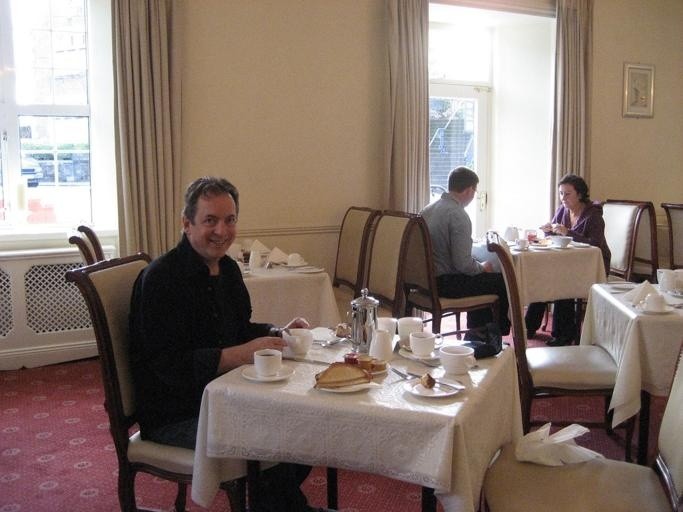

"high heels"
[546,333,580,346]
[527,329,536,339]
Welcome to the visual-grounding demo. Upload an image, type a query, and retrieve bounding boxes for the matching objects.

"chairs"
[477,345,682,510]
[485,231,637,464]
[539,201,648,334]
[63,253,282,512]
[325,207,380,306]
[340,209,419,324]
[608,201,661,283]
[68,226,101,267]
[399,212,501,348]
[661,203,683,270]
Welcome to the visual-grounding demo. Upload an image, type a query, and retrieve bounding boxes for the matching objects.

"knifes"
[406,372,466,394]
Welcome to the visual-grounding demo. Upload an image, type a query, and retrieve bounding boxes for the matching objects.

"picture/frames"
[619,62,655,121]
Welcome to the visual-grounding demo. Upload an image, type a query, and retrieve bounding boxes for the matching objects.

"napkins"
[267,247,290,264]
[621,280,655,306]
[250,240,273,257]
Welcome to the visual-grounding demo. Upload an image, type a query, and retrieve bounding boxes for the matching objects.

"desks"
[466,232,608,340]
[192,324,524,511]
[229,249,342,328]
[578,282,681,466]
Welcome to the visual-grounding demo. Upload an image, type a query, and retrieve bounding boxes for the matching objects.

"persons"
[125,177,313,510]
[524,173,612,348]
[414,167,511,360]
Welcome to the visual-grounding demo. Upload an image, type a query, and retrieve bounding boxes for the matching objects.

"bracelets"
[272,325,284,337]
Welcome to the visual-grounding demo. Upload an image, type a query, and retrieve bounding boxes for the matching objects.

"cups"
[281,328,314,356]
[367,330,395,363]
[514,229,574,247]
[253,348,283,377]
[377,316,396,342]
[397,318,424,345]
[639,267,683,313]
[440,345,475,378]
[409,330,444,357]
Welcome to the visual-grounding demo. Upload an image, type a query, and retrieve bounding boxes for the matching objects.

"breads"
[344,353,358,364]
[336,323,350,337]
[420,373,435,388]
[319,362,370,388]
[369,359,386,371]
[357,355,372,369]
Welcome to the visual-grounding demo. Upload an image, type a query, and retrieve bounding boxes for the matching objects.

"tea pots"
[346,286,381,356]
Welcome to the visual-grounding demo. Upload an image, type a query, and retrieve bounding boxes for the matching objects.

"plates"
[241,364,296,383]
[401,376,463,400]
[365,362,391,378]
[399,347,440,362]
[512,244,589,252]
[258,252,325,275]
[315,384,371,393]
